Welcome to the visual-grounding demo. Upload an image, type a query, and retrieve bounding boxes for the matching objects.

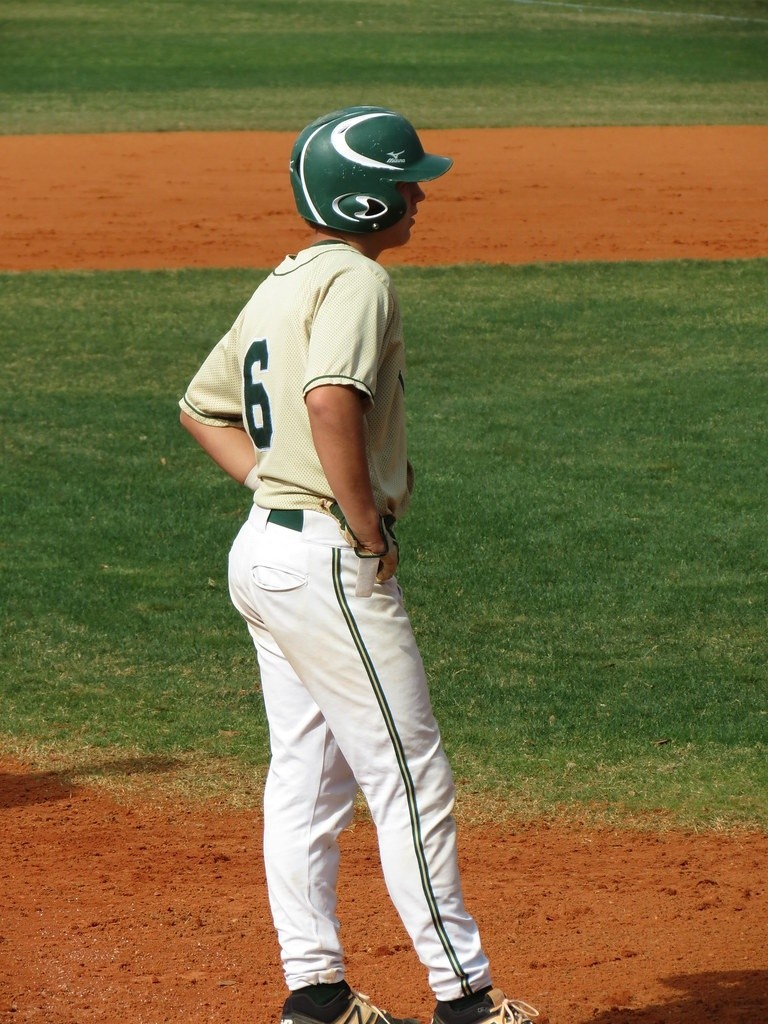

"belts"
[267,507,304,533]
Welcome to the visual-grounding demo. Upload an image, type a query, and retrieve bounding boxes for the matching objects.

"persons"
[179,105,541,1024]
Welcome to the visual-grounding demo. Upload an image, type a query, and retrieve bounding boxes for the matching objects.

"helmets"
[290,105,454,234]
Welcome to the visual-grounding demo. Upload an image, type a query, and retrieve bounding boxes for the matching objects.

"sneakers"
[432,987,539,1024]
[281,979,421,1024]
